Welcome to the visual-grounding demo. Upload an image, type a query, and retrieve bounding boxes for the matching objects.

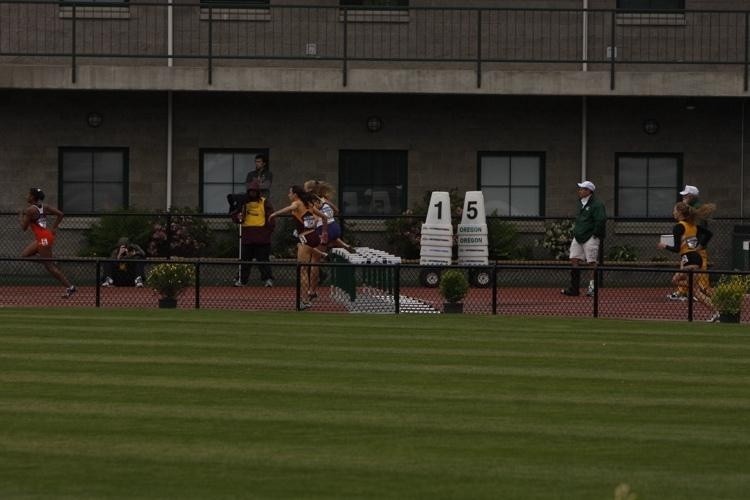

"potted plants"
[438,270,469,313]
[146,262,197,309]
[709,274,750,323]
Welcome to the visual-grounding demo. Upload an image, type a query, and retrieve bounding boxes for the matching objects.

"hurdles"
[331,243,439,314]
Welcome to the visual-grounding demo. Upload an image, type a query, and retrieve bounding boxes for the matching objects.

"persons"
[667,185,712,300]
[18,187,77,299]
[102,238,146,289]
[268,180,328,310]
[560,181,606,296]
[657,203,720,322]
[246,154,273,202]
[303,180,356,301]
[236,180,274,287]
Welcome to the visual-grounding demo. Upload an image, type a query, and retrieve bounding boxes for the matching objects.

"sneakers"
[234,281,244,287]
[134,276,144,288]
[101,277,113,287]
[62,285,75,298]
[307,291,317,302]
[585,287,595,297]
[345,245,356,253]
[300,301,312,310]
[707,313,721,323]
[668,292,688,301]
[264,278,273,287]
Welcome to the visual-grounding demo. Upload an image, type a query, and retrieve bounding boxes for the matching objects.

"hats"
[680,185,699,196]
[577,181,596,192]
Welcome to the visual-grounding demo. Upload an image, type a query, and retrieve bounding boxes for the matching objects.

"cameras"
[122,247,133,256]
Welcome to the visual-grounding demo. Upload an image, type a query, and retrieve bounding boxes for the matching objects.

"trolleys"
[413,260,498,288]
